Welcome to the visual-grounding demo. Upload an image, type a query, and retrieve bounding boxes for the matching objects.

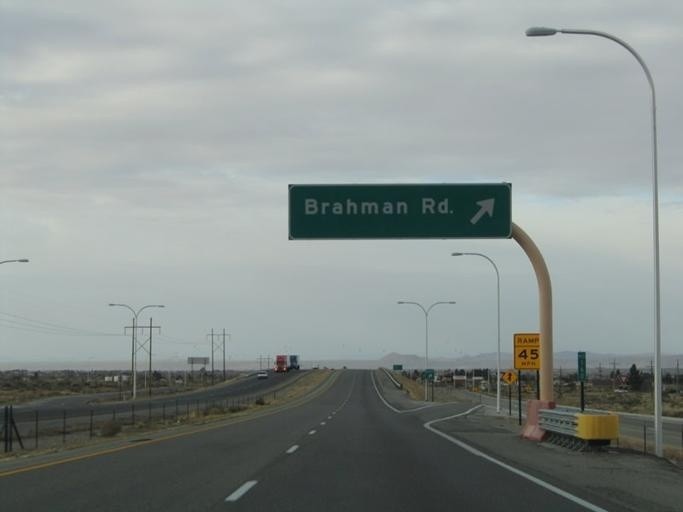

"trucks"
[289,354,299,370]
[273,355,288,372]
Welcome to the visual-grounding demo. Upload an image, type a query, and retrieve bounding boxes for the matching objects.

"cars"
[256,370,267,379]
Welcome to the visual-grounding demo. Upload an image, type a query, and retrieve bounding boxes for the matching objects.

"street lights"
[109,303,164,400]
[524,27,663,460]
[397,300,457,401]
[451,252,501,413]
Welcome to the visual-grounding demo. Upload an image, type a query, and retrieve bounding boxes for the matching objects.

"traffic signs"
[577,352,585,381]
[424,369,433,382]
[288,184,512,240]
[513,333,540,370]
